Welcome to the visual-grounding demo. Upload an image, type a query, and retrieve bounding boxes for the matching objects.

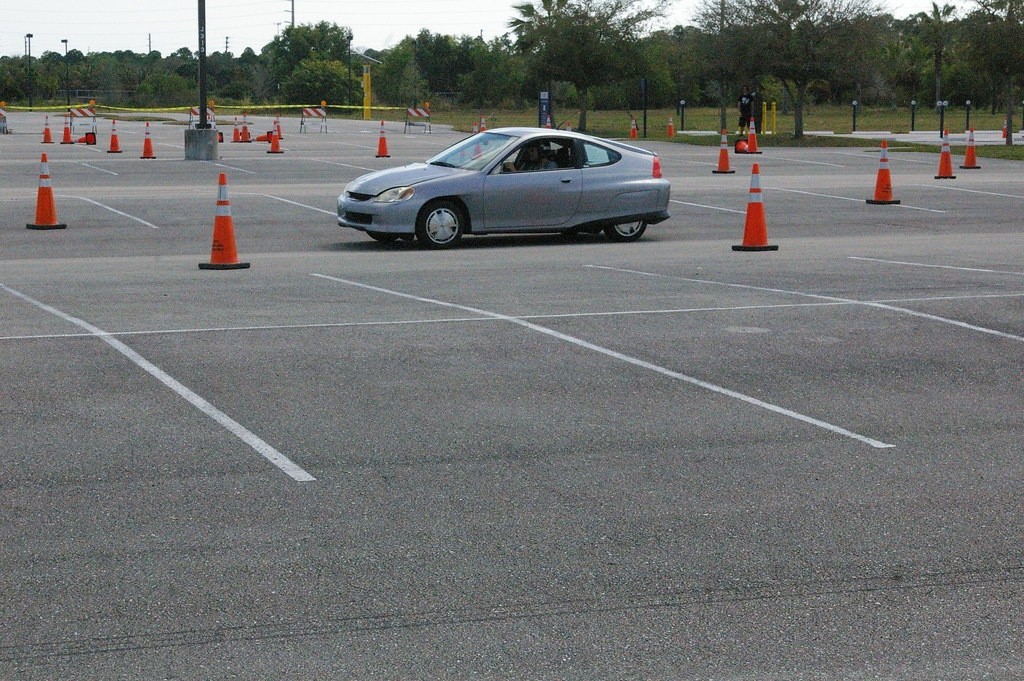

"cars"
[337,127,671,250]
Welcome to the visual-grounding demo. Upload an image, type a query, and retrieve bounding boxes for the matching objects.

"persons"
[738,85,766,137]
[505,143,557,171]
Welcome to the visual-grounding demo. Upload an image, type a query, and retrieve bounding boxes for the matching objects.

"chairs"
[554,147,569,169]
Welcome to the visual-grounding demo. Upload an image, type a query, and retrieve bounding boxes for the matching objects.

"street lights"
[61,39,70,112]
[346,35,353,115]
[27,32,33,111]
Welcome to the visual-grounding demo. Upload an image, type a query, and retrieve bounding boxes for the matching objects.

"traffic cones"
[866,141,902,204]
[267,120,285,153]
[1002,119,1007,139]
[26,153,68,229]
[141,121,156,158]
[459,141,485,162]
[199,173,250,269]
[376,119,391,158]
[75,132,96,145]
[712,126,735,174]
[60,114,74,144]
[934,130,957,179]
[473,115,486,133]
[732,162,778,251]
[959,127,982,169]
[630,118,638,141]
[546,115,551,130]
[735,116,762,155]
[565,121,572,132]
[107,118,122,153]
[667,113,674,138]
[41,115,54,143]
[231,113,283,143]
